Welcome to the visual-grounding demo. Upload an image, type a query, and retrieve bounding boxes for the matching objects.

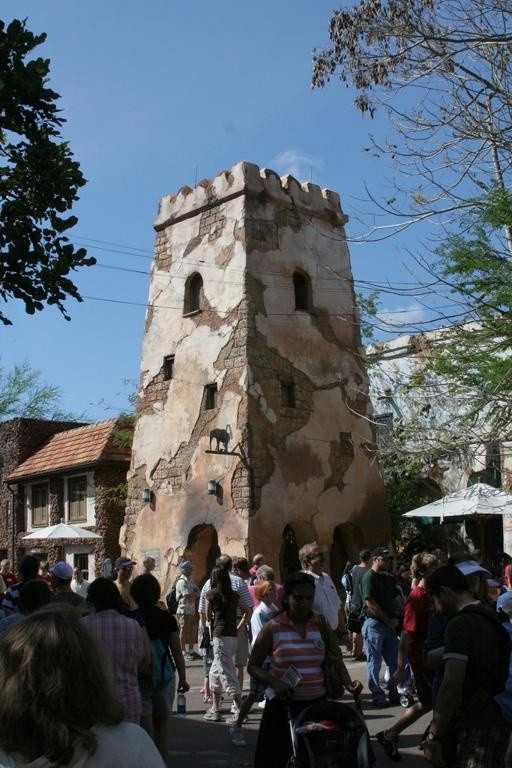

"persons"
[2,539,511,767]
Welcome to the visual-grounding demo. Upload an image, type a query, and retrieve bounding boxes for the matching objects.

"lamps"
[207,479,220,496]
[142,487,154,503]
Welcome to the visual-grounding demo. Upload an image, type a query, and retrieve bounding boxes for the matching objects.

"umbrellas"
[402,474,512,560]
[21,517,103,562]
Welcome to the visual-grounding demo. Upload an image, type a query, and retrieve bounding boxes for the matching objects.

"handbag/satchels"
[324,650,344,698]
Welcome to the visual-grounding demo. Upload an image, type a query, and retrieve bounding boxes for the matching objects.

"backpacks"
[165,578,187,614]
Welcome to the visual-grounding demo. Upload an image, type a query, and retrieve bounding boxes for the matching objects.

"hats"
[456,560,492,577]
[113,556,136,572]
[180,560,193,569]
[373,548,397,561]
[47,562,74,581]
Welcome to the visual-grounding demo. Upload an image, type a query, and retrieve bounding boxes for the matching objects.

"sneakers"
[375,730,403,761]
[200,688,250,746]
[182,650,202,662]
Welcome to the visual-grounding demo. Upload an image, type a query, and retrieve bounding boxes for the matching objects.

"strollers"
[273,682,377,768]
[391,621,419,707]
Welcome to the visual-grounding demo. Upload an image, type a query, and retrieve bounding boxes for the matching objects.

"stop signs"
[5,571,15,585]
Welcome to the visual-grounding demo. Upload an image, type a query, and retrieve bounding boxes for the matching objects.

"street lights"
[4,482,16,576]
